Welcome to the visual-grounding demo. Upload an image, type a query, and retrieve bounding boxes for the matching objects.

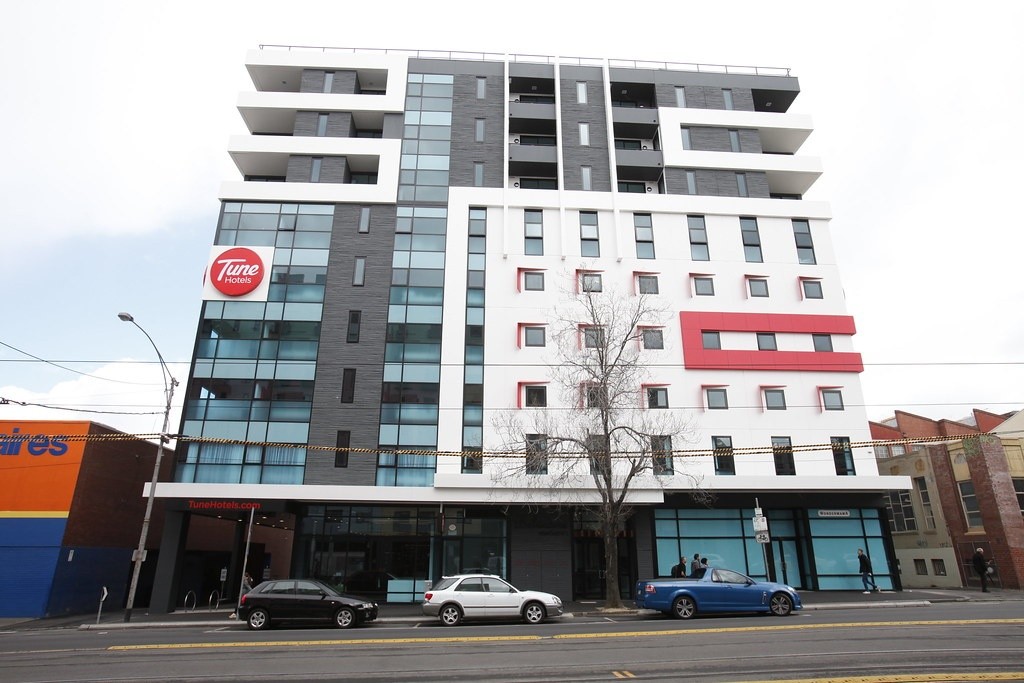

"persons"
[678,554,708,578]
[973,548,990,593]
[857,549,881,594]
[229,572,253,618]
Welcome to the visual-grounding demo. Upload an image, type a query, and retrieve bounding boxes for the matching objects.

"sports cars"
[633,567,804,620]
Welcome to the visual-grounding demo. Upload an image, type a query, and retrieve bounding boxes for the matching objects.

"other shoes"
[982,589,991,593]
[877,586,880,592]
[863,591,870,594]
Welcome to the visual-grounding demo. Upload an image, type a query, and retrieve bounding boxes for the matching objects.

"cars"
[238,578,379,631]
[422,574,563,626]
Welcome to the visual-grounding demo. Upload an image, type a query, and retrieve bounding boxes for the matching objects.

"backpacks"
[672,565,679,578]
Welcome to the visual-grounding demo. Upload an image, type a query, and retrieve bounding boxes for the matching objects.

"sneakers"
[229,613,236,619]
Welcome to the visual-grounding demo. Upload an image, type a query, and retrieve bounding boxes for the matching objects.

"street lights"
[118,312,179,624]
